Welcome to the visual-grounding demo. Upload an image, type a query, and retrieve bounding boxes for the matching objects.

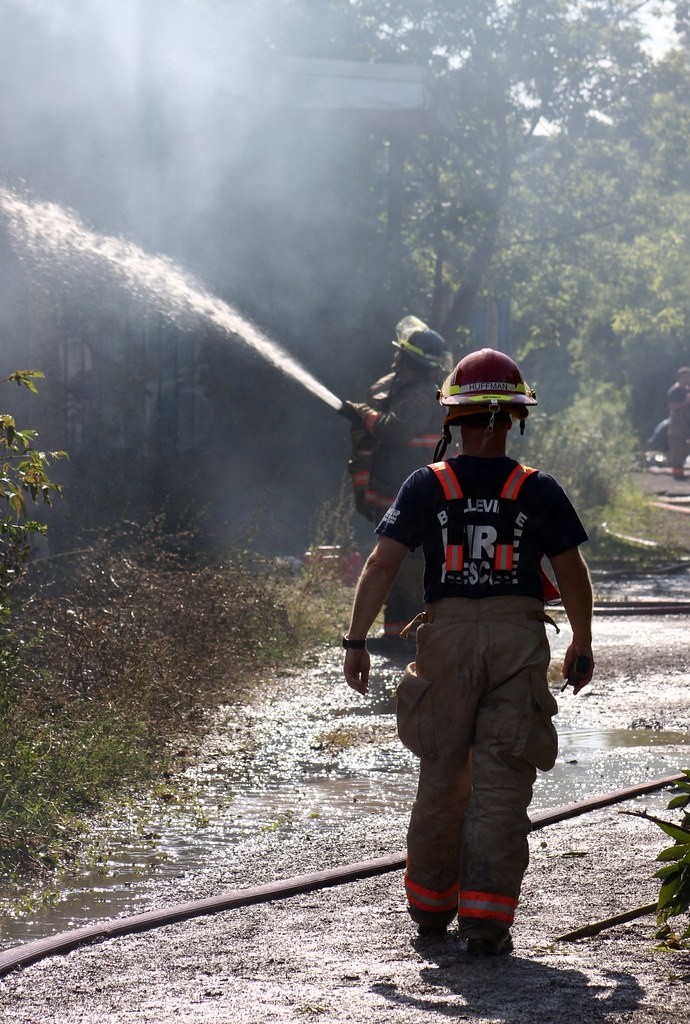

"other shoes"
[467,932,512,956]
[366,630,417,653]
[417,923,448,937]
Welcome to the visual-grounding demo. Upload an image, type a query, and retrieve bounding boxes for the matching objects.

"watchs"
[341,635,365,650]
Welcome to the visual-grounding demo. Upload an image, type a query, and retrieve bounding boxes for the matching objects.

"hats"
[441,405,529,425]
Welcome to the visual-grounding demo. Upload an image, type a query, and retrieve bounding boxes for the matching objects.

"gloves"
[345,399,374,422]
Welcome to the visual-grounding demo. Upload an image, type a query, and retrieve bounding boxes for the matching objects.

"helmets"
[391,314,455,373]
[437,346,538,410]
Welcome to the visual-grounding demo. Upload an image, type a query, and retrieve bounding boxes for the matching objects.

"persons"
[667,368,690,481]
[341,348,594,963]
[646,418,690,467]
[336,315,454,652]
